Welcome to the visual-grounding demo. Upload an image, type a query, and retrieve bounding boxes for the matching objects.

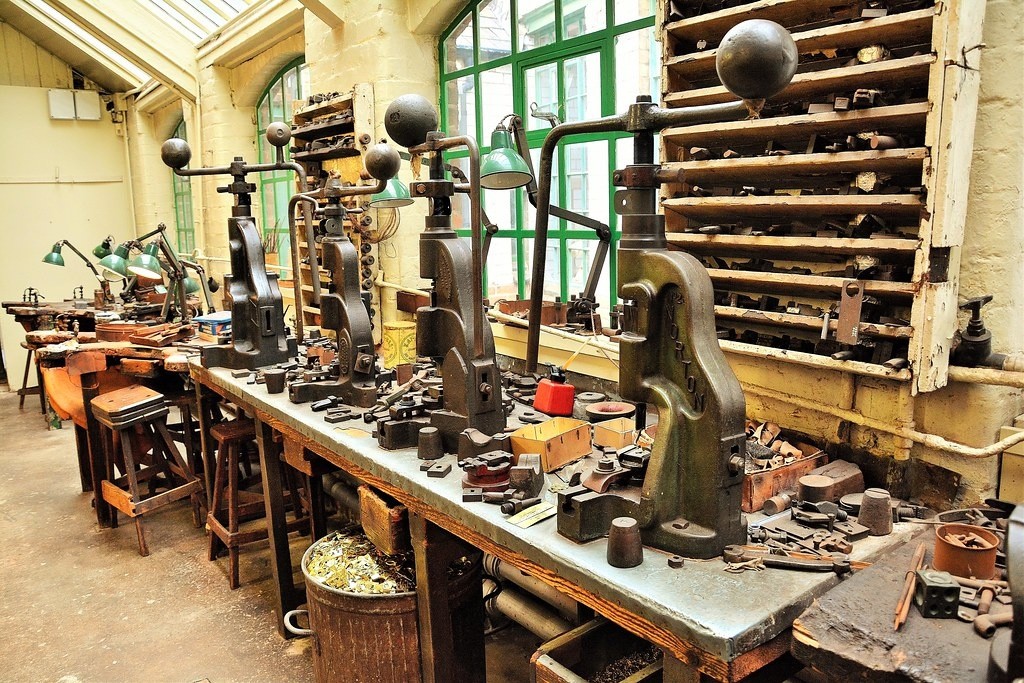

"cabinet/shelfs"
[290,81,383,345]
[652,0,989,394]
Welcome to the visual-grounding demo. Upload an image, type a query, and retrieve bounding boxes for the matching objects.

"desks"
[0,301,934,683]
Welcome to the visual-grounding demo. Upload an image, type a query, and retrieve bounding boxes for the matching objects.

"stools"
[204,417,327,588]
[148,379,224,501]
[90,385,212,559]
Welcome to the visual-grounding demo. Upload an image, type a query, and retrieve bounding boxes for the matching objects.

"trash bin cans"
[281,524,502,683]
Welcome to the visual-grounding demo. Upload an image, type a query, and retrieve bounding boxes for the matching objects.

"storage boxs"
[593,416,636,450]
[508,416,592,472]
[640,422,828,512]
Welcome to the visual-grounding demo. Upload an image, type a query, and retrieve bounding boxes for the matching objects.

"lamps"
[478,113,611,313]
[367,146,498,268]
[40,222,215,325]
